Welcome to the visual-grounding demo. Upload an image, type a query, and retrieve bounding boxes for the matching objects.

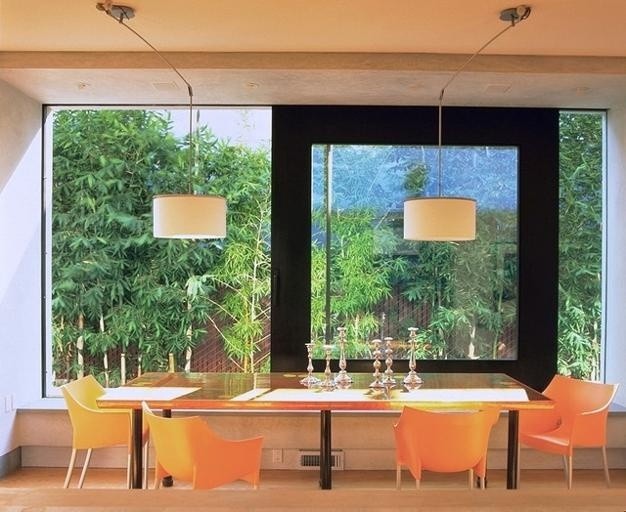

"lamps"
[99,1,226,239]
[402,4,534,244]
